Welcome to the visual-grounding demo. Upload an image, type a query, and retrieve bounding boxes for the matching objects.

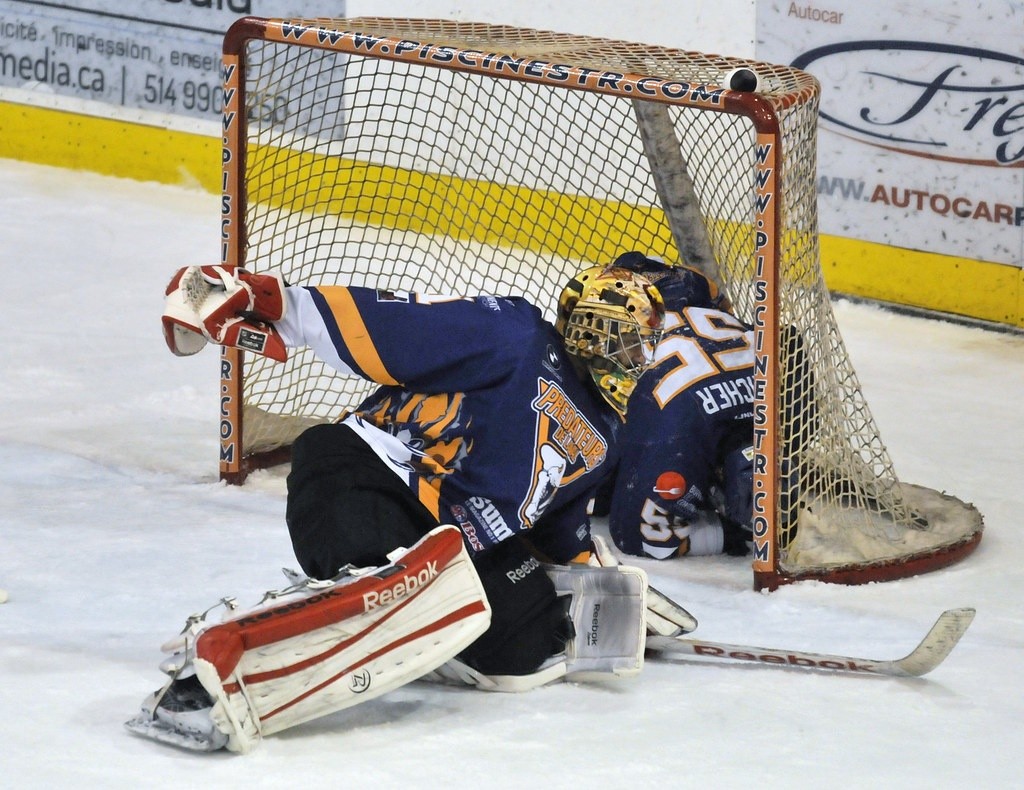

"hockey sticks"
[642,605,980,681]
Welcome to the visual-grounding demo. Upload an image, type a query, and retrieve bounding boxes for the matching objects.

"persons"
[140,266,697,746]
[581,251,818,561]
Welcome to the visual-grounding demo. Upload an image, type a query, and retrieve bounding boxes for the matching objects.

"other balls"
[728,68,758,93]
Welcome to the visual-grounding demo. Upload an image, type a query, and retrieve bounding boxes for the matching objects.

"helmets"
[553,266,666,414]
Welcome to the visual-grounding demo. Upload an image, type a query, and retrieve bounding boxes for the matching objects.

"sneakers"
[140,675,220,743]
